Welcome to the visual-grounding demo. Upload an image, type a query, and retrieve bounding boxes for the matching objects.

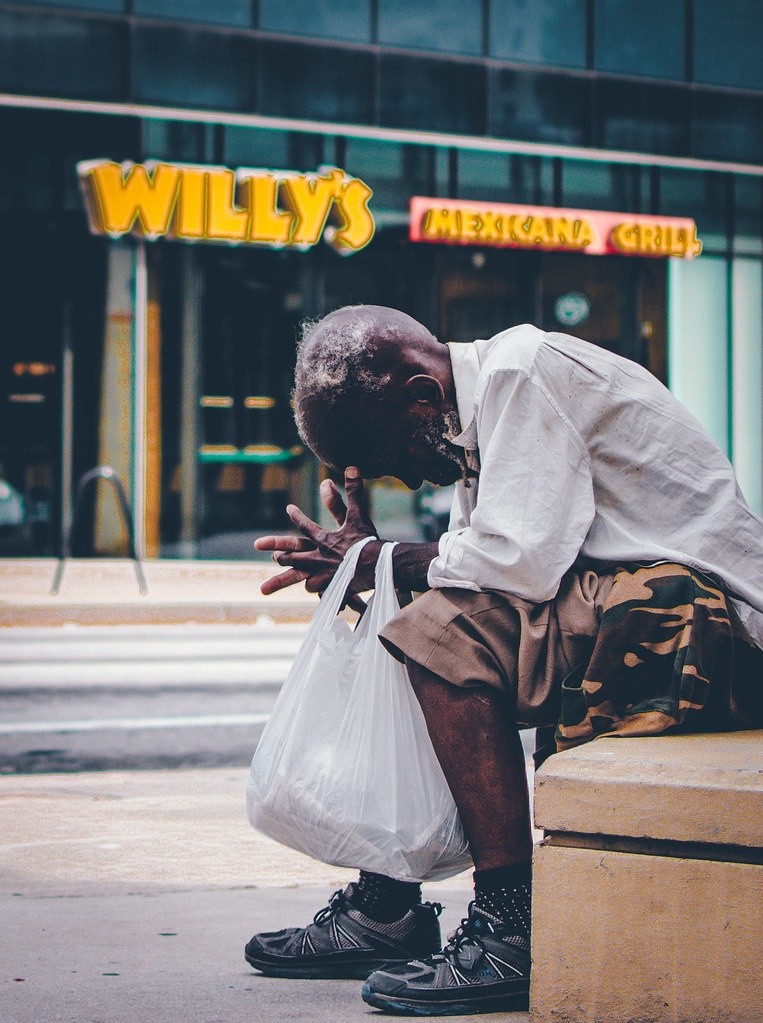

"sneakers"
[361,901,531,1016]
[244,881,442,978]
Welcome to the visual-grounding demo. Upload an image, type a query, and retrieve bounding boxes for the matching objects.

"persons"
[245,306,763,1017]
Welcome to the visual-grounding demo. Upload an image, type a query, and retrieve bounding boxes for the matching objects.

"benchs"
[529,711,763,1023]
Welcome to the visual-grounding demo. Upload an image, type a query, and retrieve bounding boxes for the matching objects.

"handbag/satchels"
[245,537,474,882]
